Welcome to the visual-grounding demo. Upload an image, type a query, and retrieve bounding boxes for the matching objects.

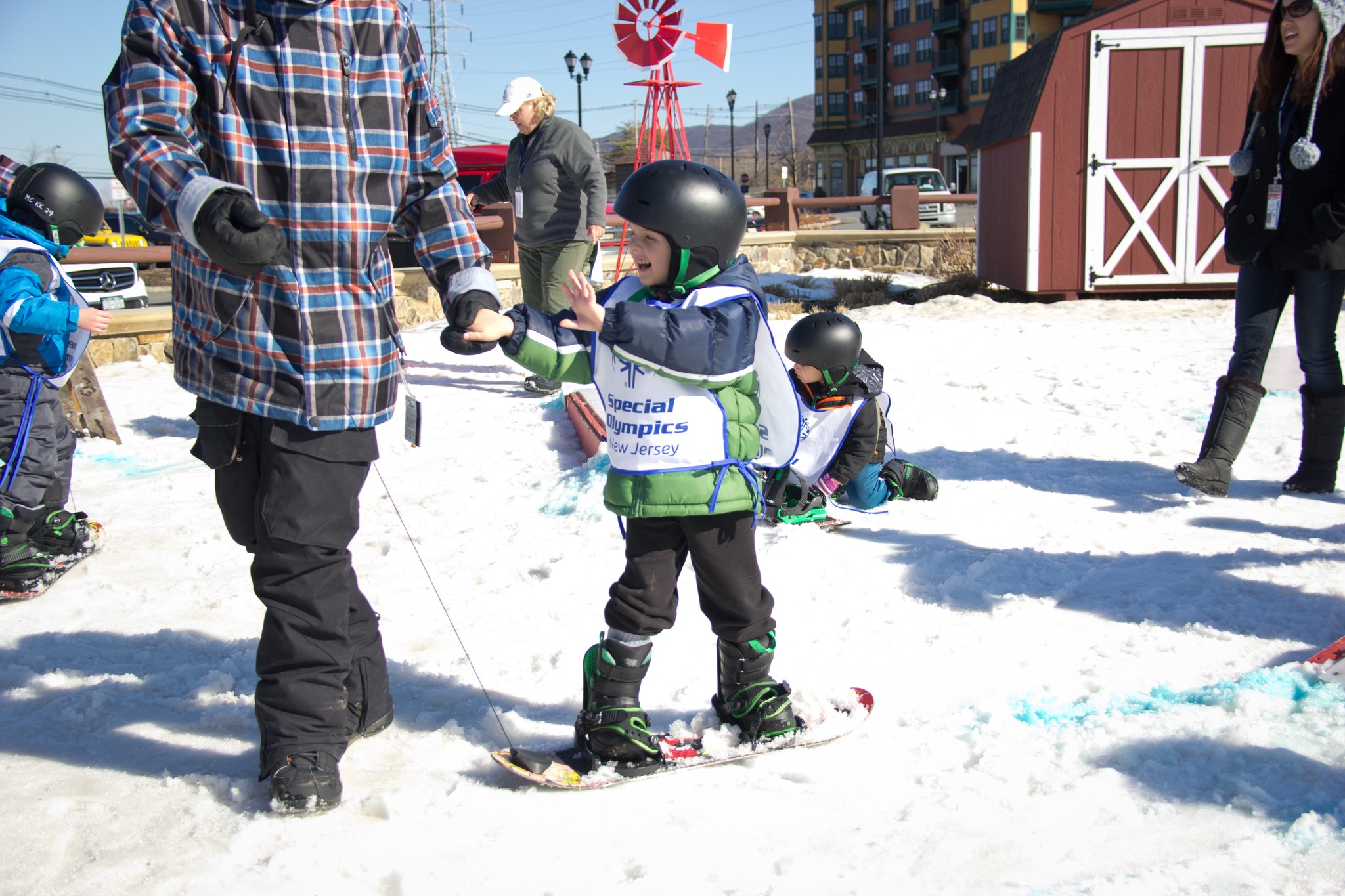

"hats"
[494,76,540,118]
[1230,0,1345,177]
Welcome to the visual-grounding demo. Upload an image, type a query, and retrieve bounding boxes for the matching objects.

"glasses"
[1281,0,1315,18]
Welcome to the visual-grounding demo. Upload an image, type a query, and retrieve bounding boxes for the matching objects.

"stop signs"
[741,174,748,183]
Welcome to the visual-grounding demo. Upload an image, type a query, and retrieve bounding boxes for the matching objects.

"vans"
[859,166,957,230]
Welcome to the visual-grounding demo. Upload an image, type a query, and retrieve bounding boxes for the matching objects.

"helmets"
[4,162,104,237]
[614,158,748,268]
[784,313,863,373]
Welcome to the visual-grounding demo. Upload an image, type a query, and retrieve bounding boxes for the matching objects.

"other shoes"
[270,751,341,814]
[348,707,396,746]
[25,506,101,555]
[525,375,563,394]
[0,514,42,580]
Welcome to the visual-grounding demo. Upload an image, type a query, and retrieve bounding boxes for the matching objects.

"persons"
[559,157,803,776]
[756,313,941,515]
[0,166,112,591]
[926,178,935,186]
[101,0,503,814]
[1177,0,1345,496]
[467,78,608,395]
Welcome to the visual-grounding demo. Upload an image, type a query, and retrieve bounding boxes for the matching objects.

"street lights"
[763,122,772,189]
[564,50,593,129]
[929,87,947,169]
[726,90,738,180]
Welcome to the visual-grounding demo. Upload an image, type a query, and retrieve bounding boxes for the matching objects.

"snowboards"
[486,685,874,787]
[0,518,109,602]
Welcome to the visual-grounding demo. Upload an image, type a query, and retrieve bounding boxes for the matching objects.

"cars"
[798,191,822,215]
[451,144,512,215]
[743,195,765,232]
[61,211,173,311]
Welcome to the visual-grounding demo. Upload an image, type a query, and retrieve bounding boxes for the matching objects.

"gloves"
[192,189,287,277]
[439,291,498,356]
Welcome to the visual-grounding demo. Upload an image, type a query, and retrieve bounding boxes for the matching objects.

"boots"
[1174,377,1267,498]
[713,635,796,738]
[1282,386,1345,493]
[582,635,654,762]
[774,486,828,522]
[879,456,939,502]
